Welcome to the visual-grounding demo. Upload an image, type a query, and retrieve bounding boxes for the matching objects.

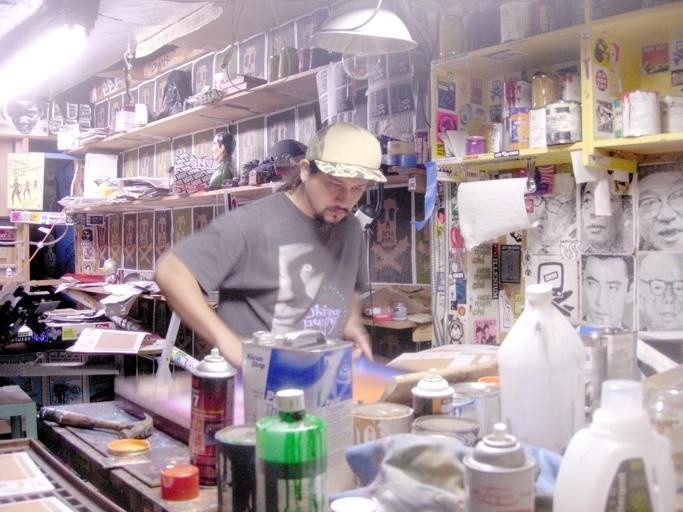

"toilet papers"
[456,178,539,251]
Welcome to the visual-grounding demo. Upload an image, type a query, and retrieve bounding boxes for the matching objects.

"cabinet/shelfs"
[429,0,683,173]
[65,56,428,330]
[0,134,57,289]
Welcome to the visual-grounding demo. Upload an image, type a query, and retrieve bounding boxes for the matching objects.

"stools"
[0,384,38,441]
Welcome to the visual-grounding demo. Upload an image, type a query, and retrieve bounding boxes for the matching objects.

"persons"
[581,254,633,329]
[81,229,93,241]
[8,176,23,205]
[636,162,682,253]
[471,324,482,343]
[579,178,636,254]
[529,171,575,255]
[121,49,134,106]
[206,131,236,187]
[150,121,388,370]
[481,325,496,343]
[6,97,44,135]
[636,253,683,331]
[19,178,31,199]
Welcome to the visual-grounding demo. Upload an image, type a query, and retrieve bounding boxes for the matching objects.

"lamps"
[311,0,418,56]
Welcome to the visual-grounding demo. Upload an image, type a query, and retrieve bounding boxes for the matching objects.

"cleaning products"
[551,379,677,512]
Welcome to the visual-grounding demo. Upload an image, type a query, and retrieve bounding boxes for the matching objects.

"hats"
[304,122,387,183]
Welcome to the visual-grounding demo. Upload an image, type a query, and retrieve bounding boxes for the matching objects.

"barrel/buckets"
[498,284,586,457]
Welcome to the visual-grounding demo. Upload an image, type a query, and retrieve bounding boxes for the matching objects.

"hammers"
[40,406,154,439]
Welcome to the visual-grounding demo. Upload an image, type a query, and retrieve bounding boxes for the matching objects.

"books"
[372,361,498,408]
[99,289,141,318]
